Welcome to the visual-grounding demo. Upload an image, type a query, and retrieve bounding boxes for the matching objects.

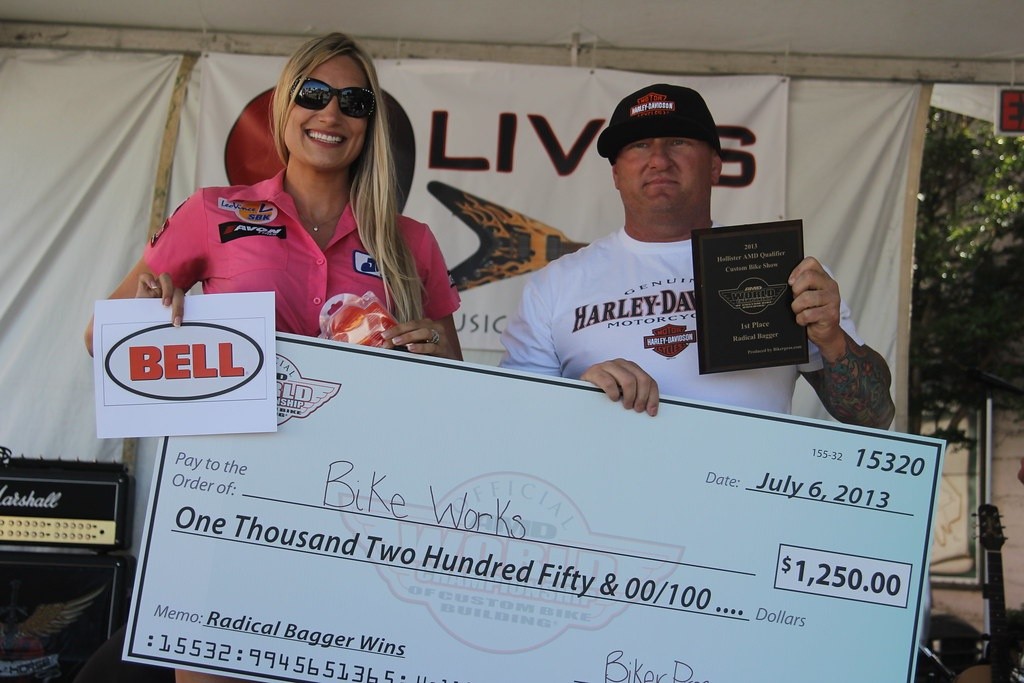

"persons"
[84,29,465,683]
[500,82,895,435]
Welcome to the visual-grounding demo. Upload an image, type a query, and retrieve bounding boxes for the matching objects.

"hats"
[597,84,723,165]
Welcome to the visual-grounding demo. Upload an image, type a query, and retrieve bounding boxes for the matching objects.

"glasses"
[289,77,376,118]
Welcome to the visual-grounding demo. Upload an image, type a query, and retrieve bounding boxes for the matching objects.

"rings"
[426,328,440,344]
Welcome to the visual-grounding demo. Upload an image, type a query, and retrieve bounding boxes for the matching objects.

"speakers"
[0,545,137,683]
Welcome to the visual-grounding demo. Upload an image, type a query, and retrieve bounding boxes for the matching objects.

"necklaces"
[296,206,339,232]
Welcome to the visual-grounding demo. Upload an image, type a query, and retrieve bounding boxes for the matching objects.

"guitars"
[425,179,601,294]
[955,501,1022,682]
